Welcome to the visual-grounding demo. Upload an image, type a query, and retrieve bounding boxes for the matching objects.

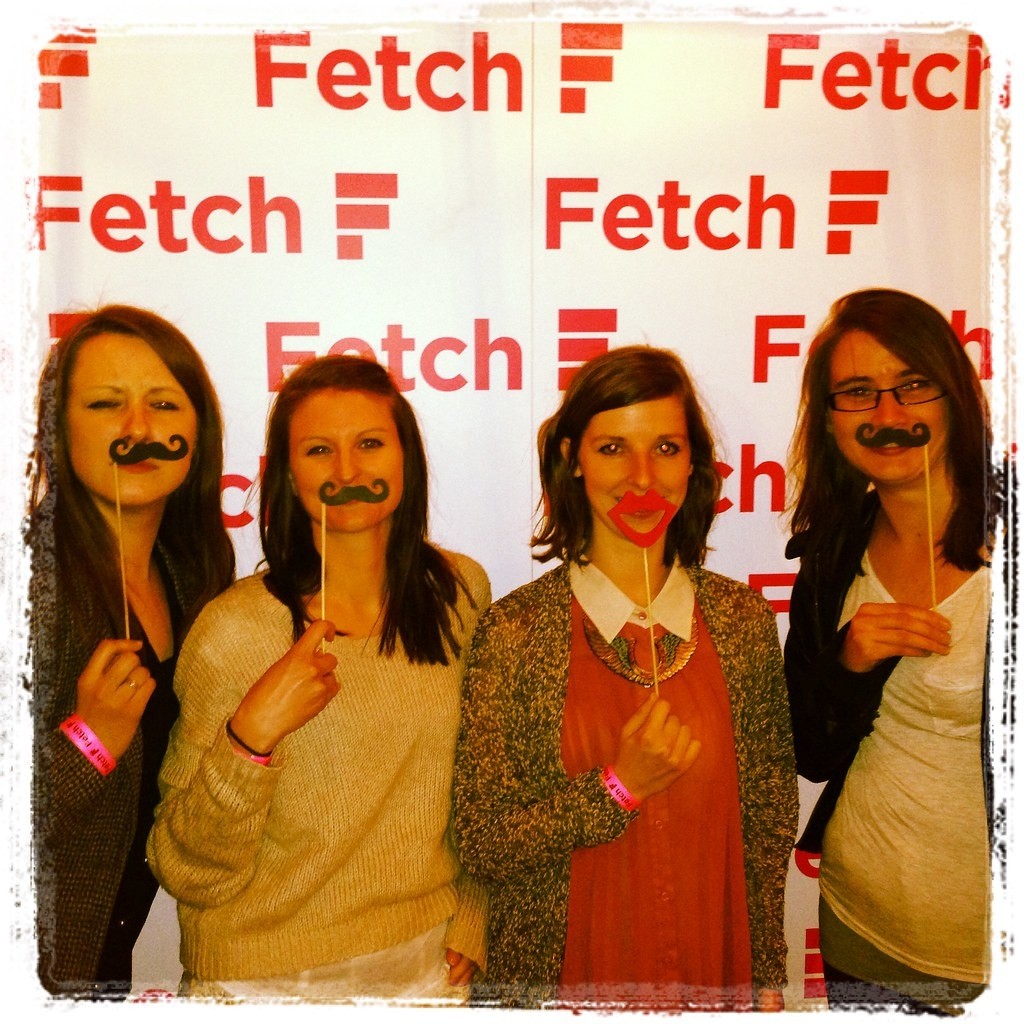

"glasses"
[826,379,947,412]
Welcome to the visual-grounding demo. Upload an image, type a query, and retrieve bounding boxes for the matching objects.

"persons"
[449,346,800,1011]
[143,355,494,1006]
[21,304,237,1001]
[782,288,997,1014]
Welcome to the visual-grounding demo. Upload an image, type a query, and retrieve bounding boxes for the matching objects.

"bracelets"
[599,766,640,815]
[60,714,116,777]
[227,723,272,766]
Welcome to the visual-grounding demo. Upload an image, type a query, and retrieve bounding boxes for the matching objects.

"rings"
[126,678,140,689]
